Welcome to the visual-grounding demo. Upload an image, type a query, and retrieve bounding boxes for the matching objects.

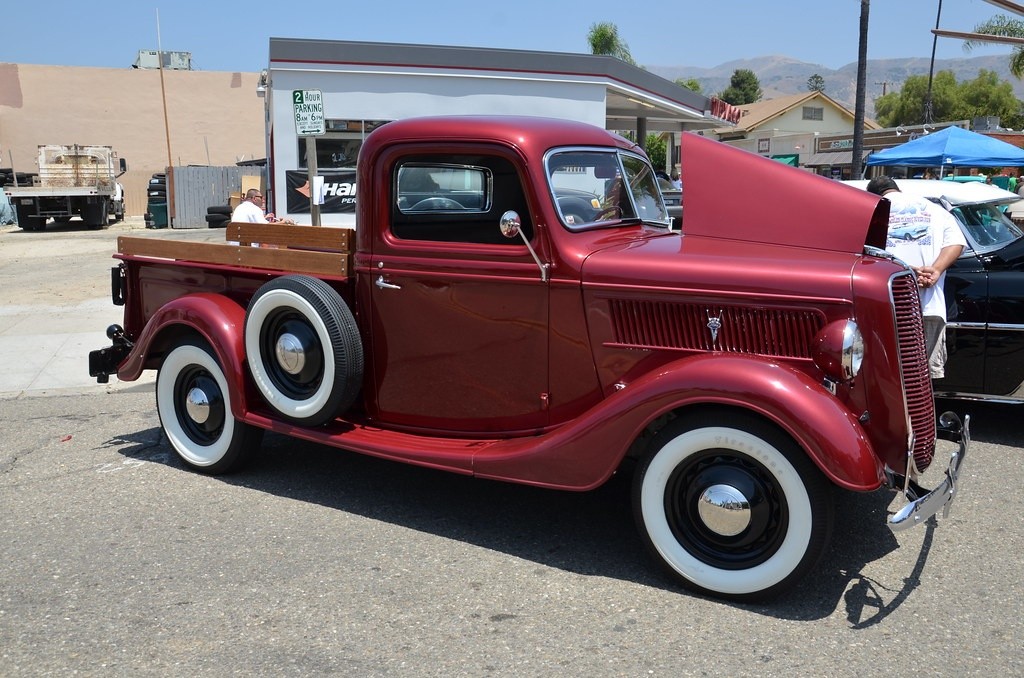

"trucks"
[5,144,128,230]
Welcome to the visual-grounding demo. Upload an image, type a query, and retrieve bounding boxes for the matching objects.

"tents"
[866,123,1024,181]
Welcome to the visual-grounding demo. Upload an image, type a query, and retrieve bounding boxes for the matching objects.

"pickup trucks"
[85,111,975,601]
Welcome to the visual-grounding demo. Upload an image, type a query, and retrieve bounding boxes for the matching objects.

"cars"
[653,176,683,221]
[838,175,1023,407]
[395,185,601,230]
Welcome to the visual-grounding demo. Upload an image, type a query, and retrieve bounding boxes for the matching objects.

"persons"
[986,177,999,186]
[1007,172,1024,199]
[226,188,295,248]
[863,174,970,380]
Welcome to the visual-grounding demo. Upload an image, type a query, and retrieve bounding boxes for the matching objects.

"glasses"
[255,195,264,200]
